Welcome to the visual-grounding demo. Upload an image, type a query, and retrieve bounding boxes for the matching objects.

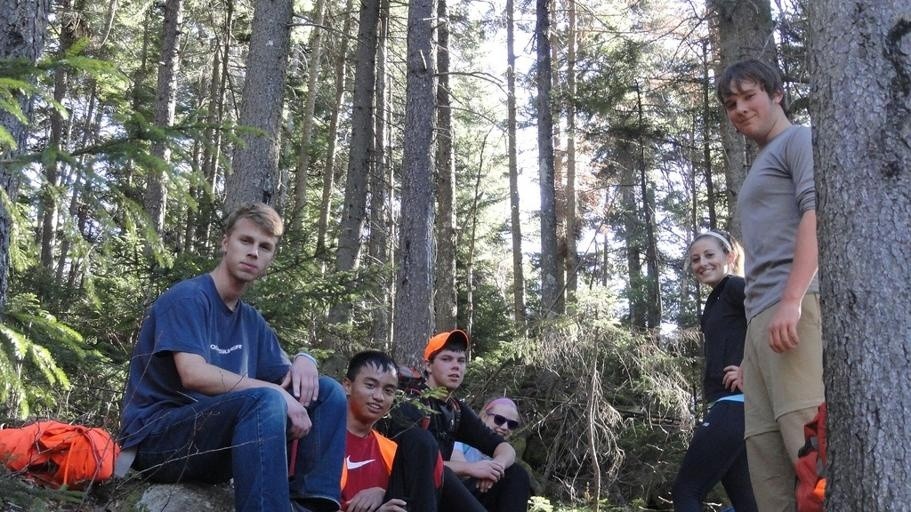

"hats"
[424,329,470,364]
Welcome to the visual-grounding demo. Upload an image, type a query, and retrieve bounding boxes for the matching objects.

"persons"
[112,198,347,512]
[715,54,820,512]
[669,227,759,511]
[448,395,521,493]
[289,350,443,512]
[387,327,516,512]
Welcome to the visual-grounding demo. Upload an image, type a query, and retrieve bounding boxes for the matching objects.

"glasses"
[488,413,518,430]
